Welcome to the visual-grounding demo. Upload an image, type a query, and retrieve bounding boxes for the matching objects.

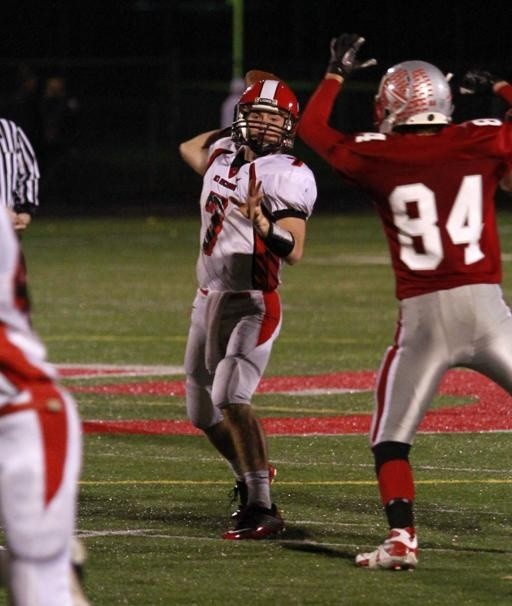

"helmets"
[374,59,453,127]
[234,78,300,122]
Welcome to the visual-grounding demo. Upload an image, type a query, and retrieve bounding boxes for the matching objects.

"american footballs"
[245,70,280,89]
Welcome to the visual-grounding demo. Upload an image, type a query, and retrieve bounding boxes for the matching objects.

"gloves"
[459,69,503,94]
[326,33,378,78]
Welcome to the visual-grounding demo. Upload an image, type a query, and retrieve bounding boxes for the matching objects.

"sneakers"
[222,464,286,539]
[355,527,419,571]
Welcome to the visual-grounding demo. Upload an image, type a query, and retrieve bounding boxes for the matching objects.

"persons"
[180,77,317,540]
[296,33,512,570]
